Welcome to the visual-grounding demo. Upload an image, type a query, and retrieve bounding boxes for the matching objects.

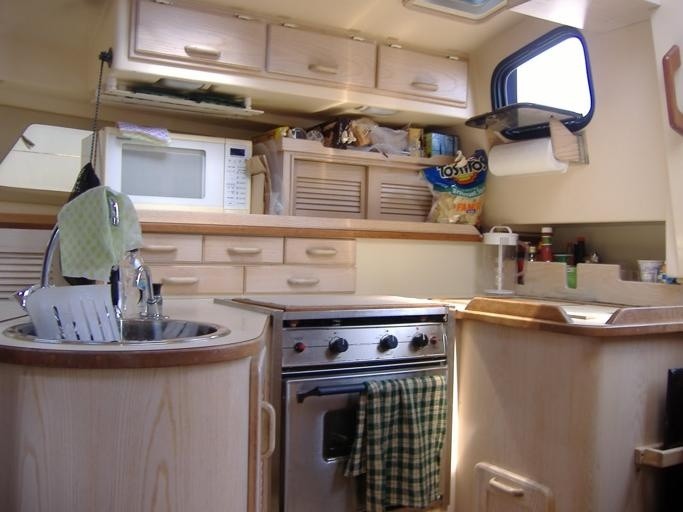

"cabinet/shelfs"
[113,2,267,102]
[125,229,356,299]
[376,21,470,131]
[269,10,377,121]
[254,138,456,222]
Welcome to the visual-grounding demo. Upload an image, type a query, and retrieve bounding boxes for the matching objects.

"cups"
[637,259,664,283]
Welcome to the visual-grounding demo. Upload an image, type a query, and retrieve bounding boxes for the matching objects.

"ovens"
[276,310,449,512]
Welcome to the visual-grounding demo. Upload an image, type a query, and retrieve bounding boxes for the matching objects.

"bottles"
[528,225,586,265]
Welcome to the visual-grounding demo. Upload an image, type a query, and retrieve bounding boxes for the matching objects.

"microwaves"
[81,126,253,213]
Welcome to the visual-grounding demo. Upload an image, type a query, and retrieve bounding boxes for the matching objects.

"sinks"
[6,317,231,344]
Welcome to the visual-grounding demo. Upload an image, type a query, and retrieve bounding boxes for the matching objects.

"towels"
[115,122,171,148]
[56,185,142,282]
[66,163,119,308]
[342,376,448,512]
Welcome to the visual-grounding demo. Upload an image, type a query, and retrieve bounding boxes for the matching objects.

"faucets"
[133,263,161,320]
[15,185,121,325]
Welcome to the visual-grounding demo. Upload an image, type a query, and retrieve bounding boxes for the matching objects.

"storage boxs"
[420,132,458,157]
[400,122,424,157]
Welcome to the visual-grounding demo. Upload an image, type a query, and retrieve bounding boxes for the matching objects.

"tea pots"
[481,225,518,296]
